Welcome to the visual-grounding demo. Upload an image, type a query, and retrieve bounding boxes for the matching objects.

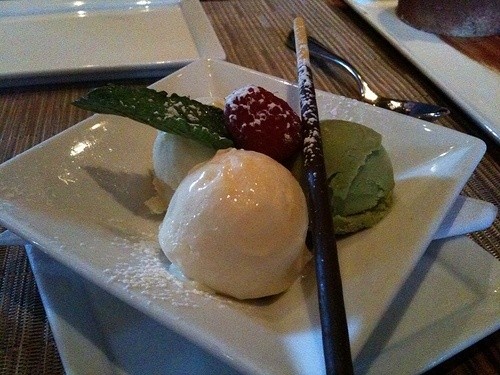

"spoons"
[285,33,450,120]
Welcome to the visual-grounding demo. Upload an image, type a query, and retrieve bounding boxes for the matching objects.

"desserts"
[65,84,395,302]
[394,0,500,37]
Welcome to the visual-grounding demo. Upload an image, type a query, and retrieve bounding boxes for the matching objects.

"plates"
[0,56,486,375]
[344,0,500,144]
[24,232,500,375]
[0,0,226,88]
